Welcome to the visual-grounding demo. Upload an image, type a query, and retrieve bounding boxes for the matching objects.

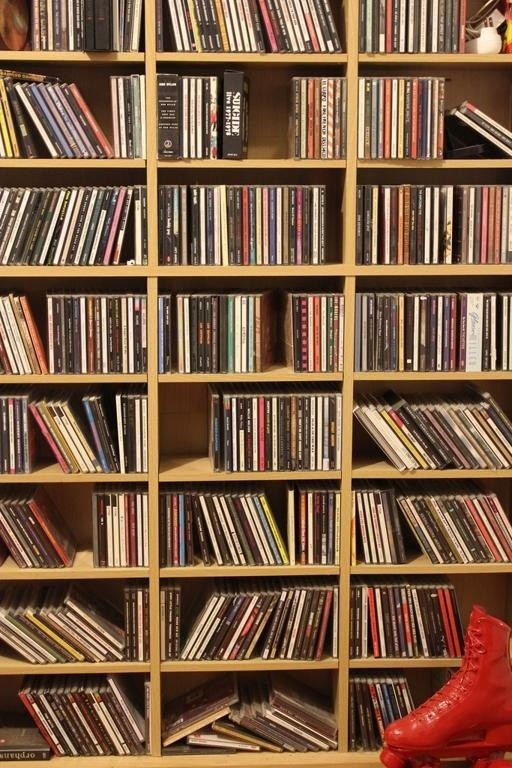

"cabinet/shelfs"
[0,1,512,768]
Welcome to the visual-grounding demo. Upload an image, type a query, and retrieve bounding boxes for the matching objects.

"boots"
[384,604,512,750]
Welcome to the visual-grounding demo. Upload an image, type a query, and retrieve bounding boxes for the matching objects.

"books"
[0,70,146,159]
[0,482,149,568]
[355,184,511,373]
[157,71,347,160]
[351,383,511,565]
[0,389,147,473]
[157,290,345,374]
[157,185,326,265]
[155,0,343,54]
[160,481,340,661]
[1,185,147,266]
[0,289,147,374]
[348,669,416,753]
[358,0,511,159]
[1,673,151,761]
[161,672,339,755]
[32,0,142,53]
[348,573,466,659]
[1,578,148,664]
[208,383,343,473]
